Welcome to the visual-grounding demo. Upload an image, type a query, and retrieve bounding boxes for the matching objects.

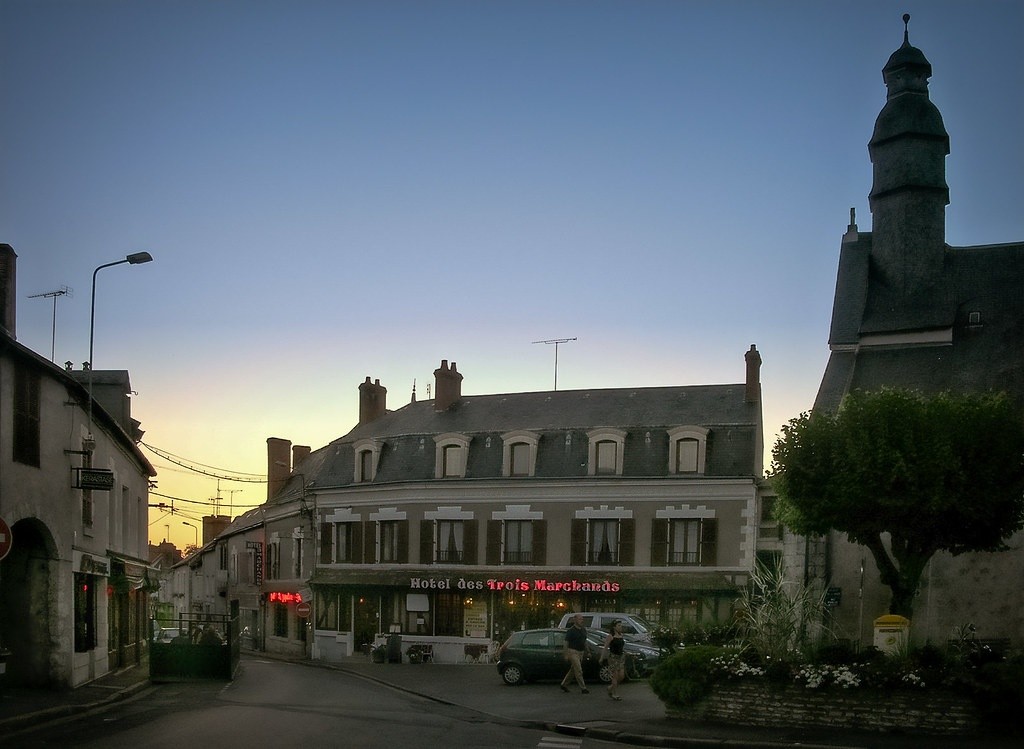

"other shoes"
[560,685,569,692]
[582,689,589,693]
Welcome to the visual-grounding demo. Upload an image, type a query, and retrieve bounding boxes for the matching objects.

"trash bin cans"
[372,645,384,663]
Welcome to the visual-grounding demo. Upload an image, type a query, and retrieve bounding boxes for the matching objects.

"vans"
[559,611,686,653]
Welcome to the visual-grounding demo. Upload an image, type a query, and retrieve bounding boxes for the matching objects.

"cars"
[153,620,188,644]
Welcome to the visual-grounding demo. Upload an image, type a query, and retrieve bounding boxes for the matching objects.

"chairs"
[421,643,434,664]
[453,639,502,665]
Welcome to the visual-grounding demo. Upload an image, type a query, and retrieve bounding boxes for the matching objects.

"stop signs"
[296,602,311,618]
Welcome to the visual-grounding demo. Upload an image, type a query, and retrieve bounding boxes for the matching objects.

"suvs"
[497,627,671,686]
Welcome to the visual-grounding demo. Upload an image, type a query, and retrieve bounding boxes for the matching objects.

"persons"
[157,629,167,643]
[599,620,626,701]
[559,614,591,694]
[193,623,223,646]
[172,629,188,644]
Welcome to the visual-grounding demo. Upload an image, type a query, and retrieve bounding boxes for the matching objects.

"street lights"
[183,521,198,552]
[86,251,155,441]
[276,460,306,579]
[165,525,169,543]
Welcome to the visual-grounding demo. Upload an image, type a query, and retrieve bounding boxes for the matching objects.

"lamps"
[63,432,97,456]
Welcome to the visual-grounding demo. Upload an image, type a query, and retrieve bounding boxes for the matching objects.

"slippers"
[607,687,613,698]
[613,696,622,701]
